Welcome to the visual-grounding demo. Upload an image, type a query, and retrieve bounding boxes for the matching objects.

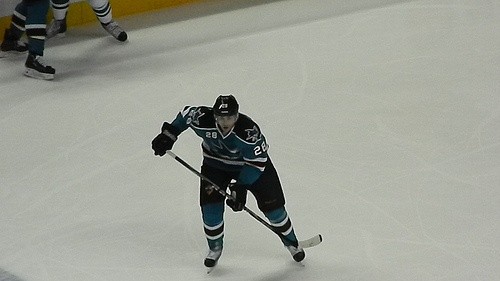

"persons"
[152,94,306,274]
[47,0,127,42]
[0,0,55,80]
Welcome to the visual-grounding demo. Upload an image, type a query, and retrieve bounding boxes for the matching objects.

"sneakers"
[97,17,129,44]
[46,10,68,40]
[286,243,306,266]
[0,28,29,58]
[204,247,223,273]
[24,53,56,79]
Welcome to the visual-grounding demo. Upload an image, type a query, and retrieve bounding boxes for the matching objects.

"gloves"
[226,182,247,211]
[152,122,180,156]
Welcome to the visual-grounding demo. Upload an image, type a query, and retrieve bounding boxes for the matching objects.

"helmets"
[212,95,239,116]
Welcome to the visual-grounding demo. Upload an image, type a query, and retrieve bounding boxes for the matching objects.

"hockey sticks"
[166,149,323,248]
